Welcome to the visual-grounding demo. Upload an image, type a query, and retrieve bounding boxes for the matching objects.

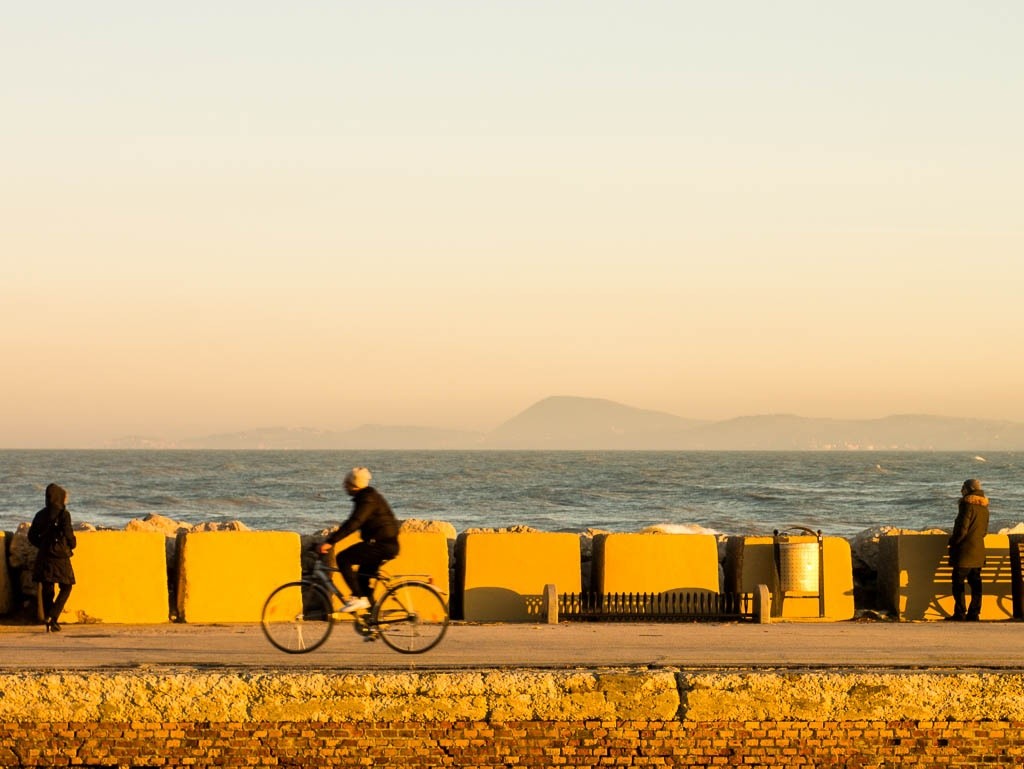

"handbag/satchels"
[49,509,72,558]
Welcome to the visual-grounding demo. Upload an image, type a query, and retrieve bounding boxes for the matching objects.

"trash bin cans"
[779,543,820,592]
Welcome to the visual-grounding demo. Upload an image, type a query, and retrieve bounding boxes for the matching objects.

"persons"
[317,466,401,630]
[27,483,77,633]
[943,478,990,622]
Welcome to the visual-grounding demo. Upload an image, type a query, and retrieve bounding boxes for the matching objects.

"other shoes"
[945,613,966,622]
[965,612,979,621]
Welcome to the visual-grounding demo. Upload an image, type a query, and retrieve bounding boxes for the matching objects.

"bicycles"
[260,541,453,656]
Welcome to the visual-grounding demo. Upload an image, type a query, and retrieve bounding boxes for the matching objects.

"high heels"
[45,616,61,632]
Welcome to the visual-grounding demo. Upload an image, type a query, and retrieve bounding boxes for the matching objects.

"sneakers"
[339,596,372,612]
[363,619,391,642]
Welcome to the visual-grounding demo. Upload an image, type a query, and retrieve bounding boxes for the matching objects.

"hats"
[963,479,980,492]
[344,467,372,488]
